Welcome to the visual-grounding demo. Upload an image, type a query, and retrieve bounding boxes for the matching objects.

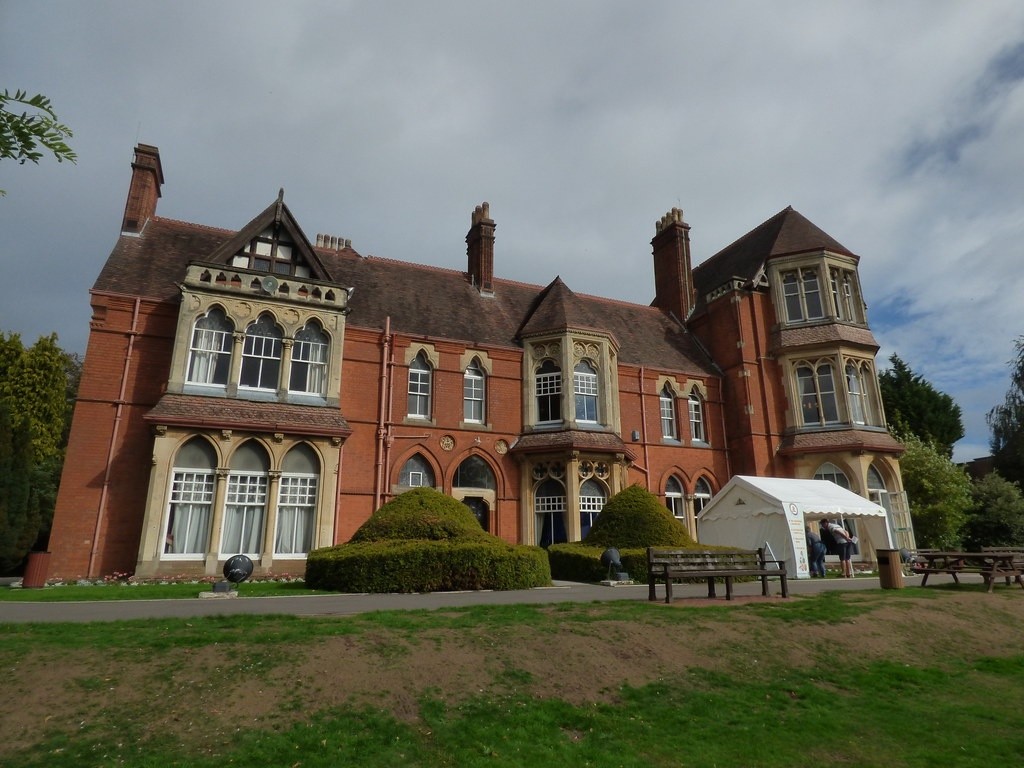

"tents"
[695,475,906,581]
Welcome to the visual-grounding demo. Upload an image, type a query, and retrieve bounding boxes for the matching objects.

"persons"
[819,518,853,579]
[804,527,826,577]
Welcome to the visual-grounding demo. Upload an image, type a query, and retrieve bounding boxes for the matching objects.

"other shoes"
[846,575,850,578]
[836,574,845,579]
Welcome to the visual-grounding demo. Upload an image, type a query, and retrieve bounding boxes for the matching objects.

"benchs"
[981,545,1024,564]
[909,566,999,578]
[900,549,958,575]
[646,547,789,604]
[953,564,1024,575]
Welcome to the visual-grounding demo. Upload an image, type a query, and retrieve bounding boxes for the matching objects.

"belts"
[812,541,823,545]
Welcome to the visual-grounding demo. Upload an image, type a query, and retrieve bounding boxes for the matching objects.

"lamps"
[213,555,254,593]
[261,275,280,293]
[600,546,629,582]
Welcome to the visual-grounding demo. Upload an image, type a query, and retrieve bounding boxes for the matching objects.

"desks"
[917,552,1024,593]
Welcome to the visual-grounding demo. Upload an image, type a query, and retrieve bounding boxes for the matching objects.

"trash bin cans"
[875,548,904,589]
